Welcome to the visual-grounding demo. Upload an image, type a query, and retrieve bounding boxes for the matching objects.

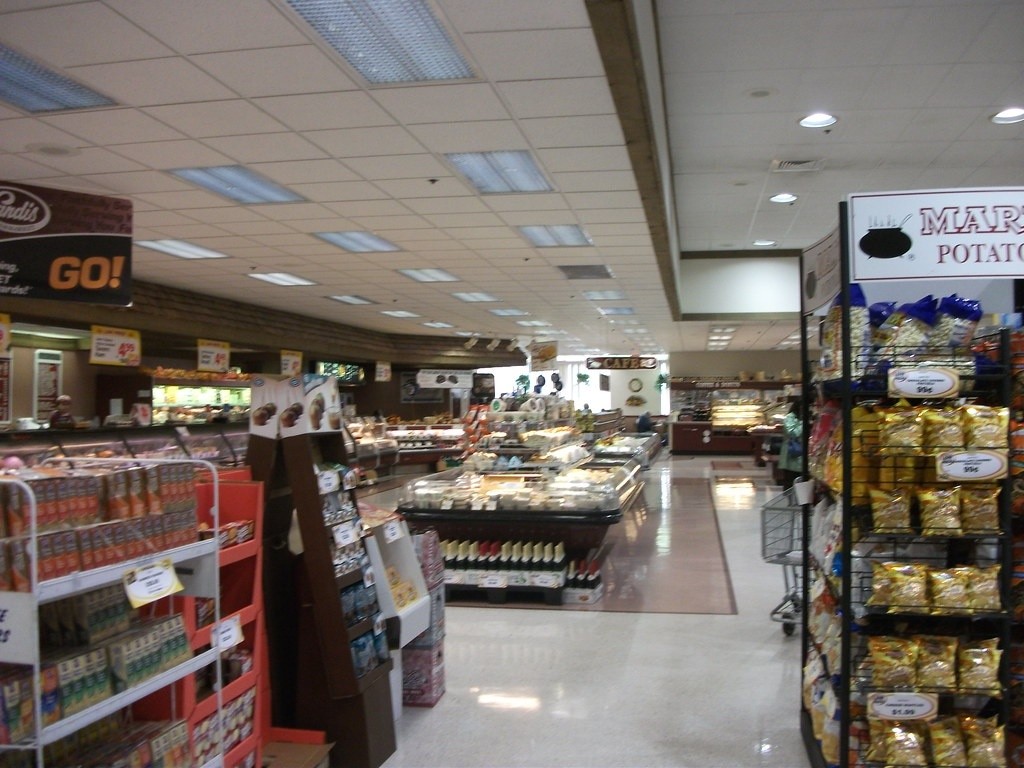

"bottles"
[435,537,605,605]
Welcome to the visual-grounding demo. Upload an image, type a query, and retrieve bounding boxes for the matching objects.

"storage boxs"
[396,531,446,709]
[0,462,252,768]
[561,583,604,604]
[443,569,568,589]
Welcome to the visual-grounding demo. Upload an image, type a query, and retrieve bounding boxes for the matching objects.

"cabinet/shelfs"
[136,465,272,768]
[1,457,224,768]
[246,429,397,768]
[356,427,466,472]
[800,198,1024,768]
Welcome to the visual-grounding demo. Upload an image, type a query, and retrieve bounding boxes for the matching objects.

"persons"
[49,395,77,427]
[778,401,802,487]
[636,412,657,433]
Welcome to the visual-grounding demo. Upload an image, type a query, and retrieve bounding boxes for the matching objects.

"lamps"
[463,335,479,351]
[506,339,519,353]
[524,339,537,351]
[486,338,502,352]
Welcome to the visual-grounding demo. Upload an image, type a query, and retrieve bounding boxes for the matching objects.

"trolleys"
[760,475,807,635]
